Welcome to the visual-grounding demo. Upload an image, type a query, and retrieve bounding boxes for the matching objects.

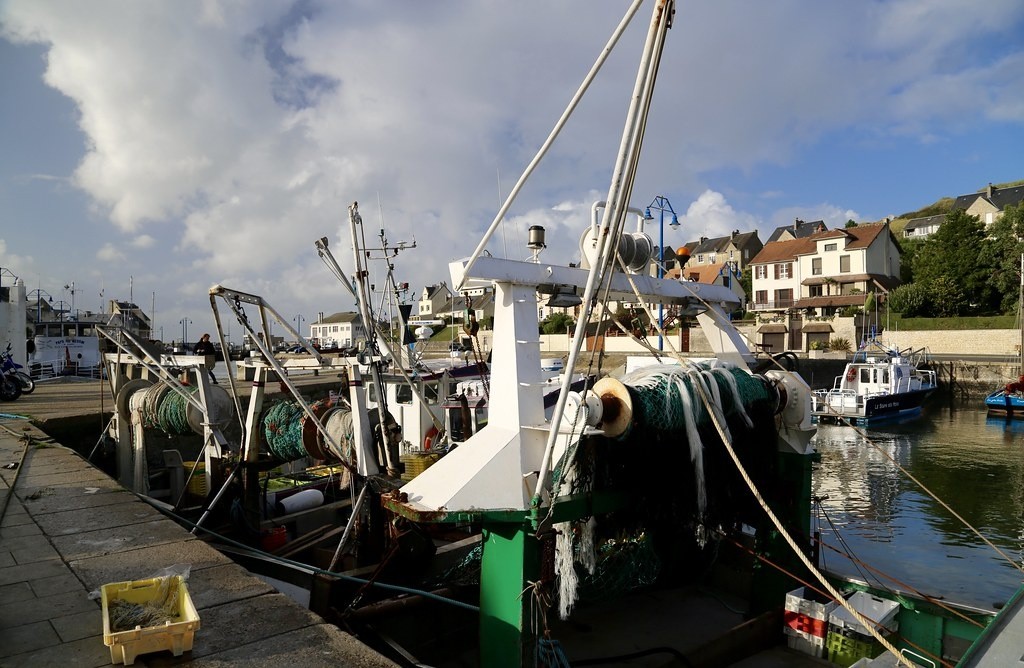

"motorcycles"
[0,343,36,403]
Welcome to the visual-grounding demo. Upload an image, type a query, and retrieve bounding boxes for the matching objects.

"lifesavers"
[847,368,857,382]
[424,427,441,450]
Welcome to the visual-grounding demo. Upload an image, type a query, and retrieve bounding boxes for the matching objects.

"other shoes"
[213,381,218,384]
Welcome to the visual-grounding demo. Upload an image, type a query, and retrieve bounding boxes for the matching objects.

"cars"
[286,342,321,354]
[339,348,358,357]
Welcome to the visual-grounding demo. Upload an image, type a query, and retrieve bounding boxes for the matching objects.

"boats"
[810,288,955,430]
[90,3,1024,668]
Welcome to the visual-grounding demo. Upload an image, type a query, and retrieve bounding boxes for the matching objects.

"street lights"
[26,288,54,322]
[51,300,72,323]
[243,320,251,335]
[180,317,193,344]
[268,318,276,335]
[293,314,305,333]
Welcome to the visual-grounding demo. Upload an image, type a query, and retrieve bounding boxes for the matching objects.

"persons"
[193,333,219,385]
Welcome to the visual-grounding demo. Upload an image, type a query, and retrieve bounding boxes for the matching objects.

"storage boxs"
[783,585,900,667]
[101,574,201,665]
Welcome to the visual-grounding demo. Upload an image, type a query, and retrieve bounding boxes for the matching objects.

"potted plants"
[808,336,852,360]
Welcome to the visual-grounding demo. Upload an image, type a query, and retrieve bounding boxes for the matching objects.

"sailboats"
[985,255,1024,424]
